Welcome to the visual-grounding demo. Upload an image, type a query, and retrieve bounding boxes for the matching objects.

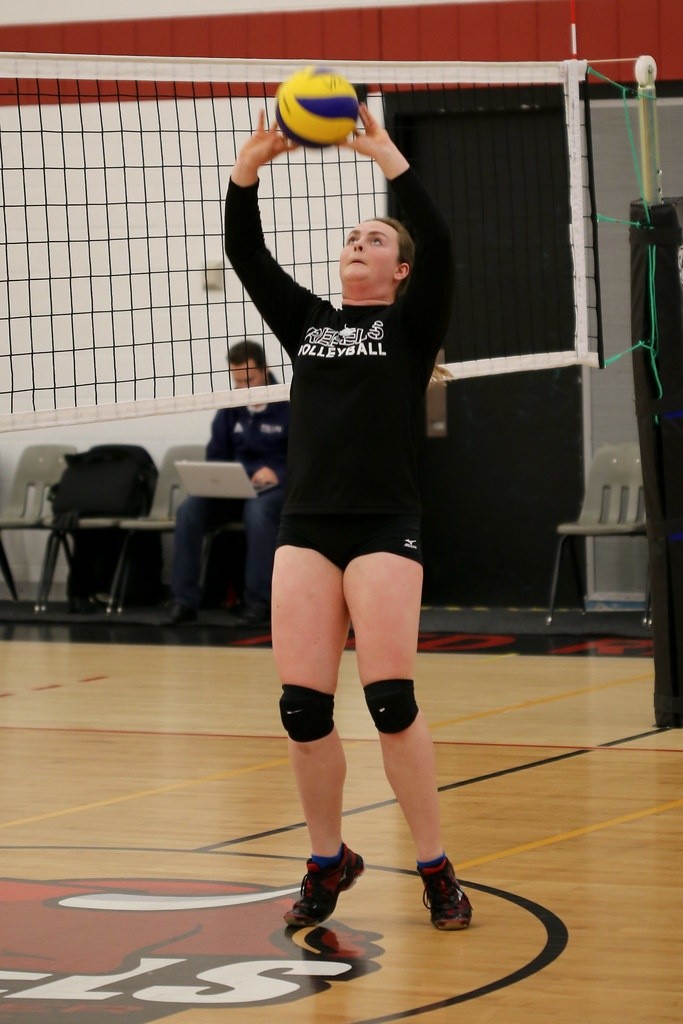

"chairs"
[0,441,246,617]
[543,440,653,626]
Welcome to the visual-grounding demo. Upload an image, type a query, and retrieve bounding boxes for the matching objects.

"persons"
[162,339,290,629]
[224,102,474,930]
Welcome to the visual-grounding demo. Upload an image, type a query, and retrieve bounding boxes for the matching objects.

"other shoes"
[160,604,198,628]
[236,609,269,627]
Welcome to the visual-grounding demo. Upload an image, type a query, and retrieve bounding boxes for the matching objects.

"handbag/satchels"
[48,444,158,519]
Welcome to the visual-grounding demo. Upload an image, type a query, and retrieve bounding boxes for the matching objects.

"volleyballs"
[271,64,358,150]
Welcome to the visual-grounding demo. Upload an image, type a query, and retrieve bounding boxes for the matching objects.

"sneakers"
[417,856,473,930]
[283,843,364,927]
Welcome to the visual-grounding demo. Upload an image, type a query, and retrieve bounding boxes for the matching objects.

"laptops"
[175,462,276,500]
